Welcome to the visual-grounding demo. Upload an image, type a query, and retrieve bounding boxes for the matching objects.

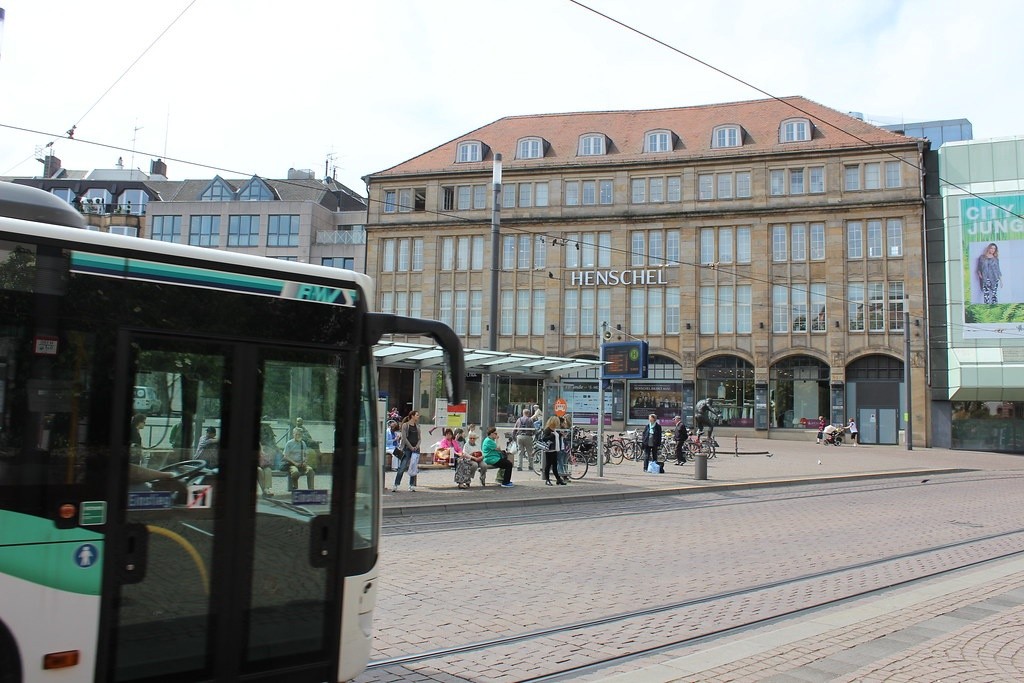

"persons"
[128,417,318,497]
[672,415,688,466]
[817,415,824,444]
[843,418,858,447]
[694,397,721,439]
[385,404,572,492]
[634,392,680,408]
[642,414,662,472]
[977,243,1005,303]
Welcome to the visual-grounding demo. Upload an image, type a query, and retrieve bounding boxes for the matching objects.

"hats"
[672,415,681,420]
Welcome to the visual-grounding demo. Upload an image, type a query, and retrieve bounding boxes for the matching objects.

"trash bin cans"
[899,431,905,445]
[694,454,708,479]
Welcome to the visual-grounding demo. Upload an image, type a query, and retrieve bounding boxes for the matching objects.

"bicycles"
[506,427,715,466]
[532,444,589,479]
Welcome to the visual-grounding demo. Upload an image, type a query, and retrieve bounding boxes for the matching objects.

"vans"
[132,385,163,416]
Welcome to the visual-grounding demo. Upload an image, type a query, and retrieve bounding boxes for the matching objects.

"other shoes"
[529,467,533,470]
[519,468,523,471]
[408,486,416,492]
[674,462,684,466]
[458,485,469,489]
[501,482,514,487]
[480,477,485,486]
[391,484,397,492]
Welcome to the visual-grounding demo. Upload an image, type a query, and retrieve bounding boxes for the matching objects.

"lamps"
[617,324,621,331]
[686,322,690,329]
[487,324,489,330]
[759,322,763,329]
[836,321,839,327]
[550,325,555,330]
[914,320,919,326]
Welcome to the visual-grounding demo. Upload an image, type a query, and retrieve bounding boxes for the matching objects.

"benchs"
[136,448,456,472]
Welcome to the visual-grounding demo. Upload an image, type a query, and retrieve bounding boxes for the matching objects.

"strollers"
[823,425,845,447]
[386,411,401,427]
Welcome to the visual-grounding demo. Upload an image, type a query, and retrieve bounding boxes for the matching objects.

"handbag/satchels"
[408,450,420,476]
[278,460,289,471]
[647,459,665,474]
[393,447,405,461]
[535,433,553,452]
[471,451,482,462]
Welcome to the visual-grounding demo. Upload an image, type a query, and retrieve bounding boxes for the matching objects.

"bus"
[0,180,467,683]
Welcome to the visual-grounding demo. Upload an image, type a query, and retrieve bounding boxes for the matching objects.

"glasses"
[469,438,476,439]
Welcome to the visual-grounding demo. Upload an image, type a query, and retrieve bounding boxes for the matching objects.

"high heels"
[556,479,566,485]
[545,480,552,486]
